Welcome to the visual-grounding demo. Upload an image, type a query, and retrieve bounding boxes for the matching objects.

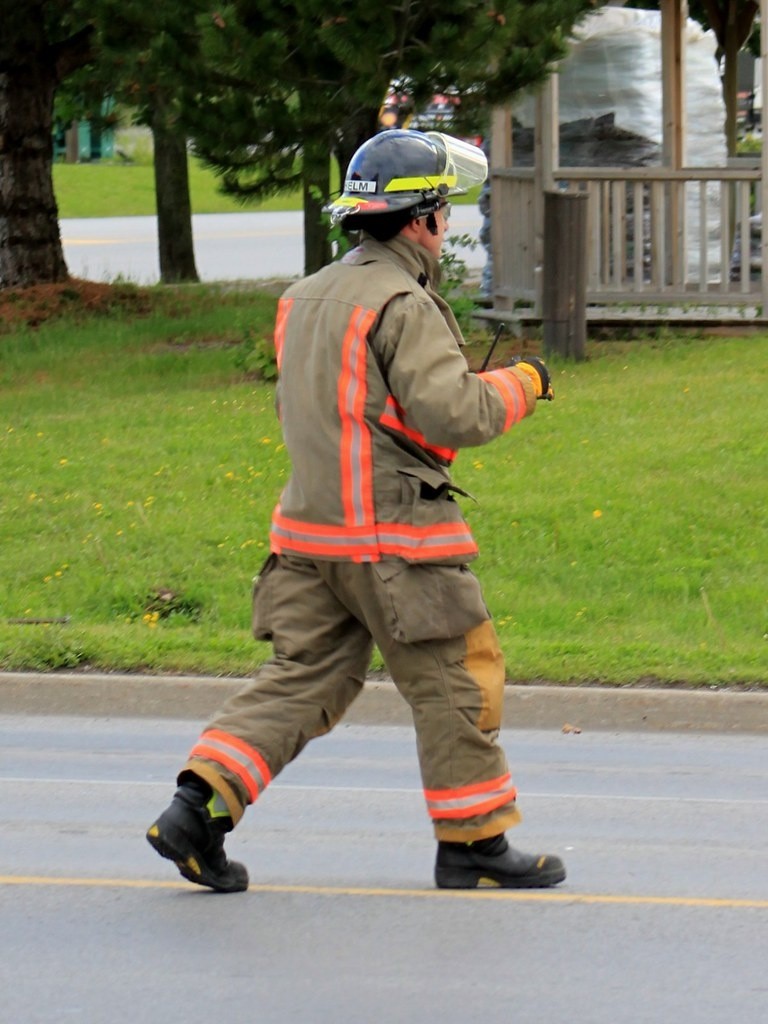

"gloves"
[513,357,555,402]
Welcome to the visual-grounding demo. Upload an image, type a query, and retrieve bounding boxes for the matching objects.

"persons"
[144,129,568,891]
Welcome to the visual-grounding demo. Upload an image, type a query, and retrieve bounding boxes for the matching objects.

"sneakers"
[146,790,249,894]
[434,845,567,891]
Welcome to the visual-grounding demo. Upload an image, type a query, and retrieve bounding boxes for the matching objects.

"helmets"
[322,128,460,228]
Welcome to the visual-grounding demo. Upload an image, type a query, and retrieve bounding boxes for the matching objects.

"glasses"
[438,201,451,220]
